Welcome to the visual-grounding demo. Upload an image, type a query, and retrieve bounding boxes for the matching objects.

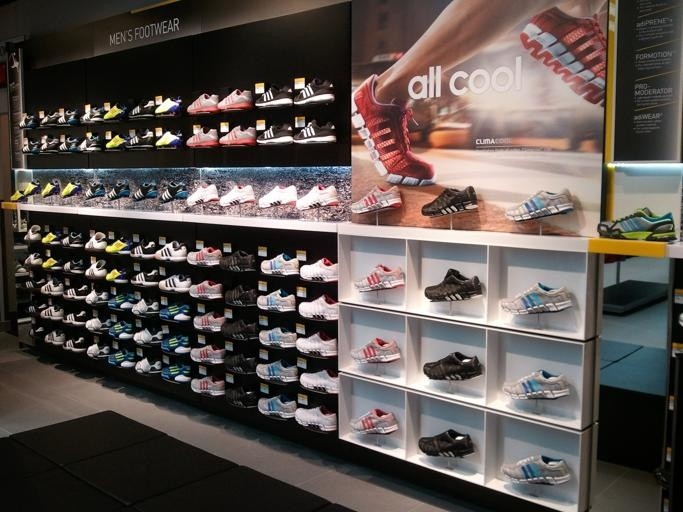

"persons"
[352,1,607,187]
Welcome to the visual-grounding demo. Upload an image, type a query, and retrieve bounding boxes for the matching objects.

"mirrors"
[589,254,671,512]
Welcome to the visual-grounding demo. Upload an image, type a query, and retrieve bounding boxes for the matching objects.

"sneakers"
[185,77,337,149]
[105,179,130,201]
[350,184,402,213]
[421,186,479,216]
[220,250,338,434]
[23,181,40,196]
[351,74,436,187]
[61,181,82,198]
[131,182,158,201]
[501,454,570,485]
[77,180,105,201]
[296,183,339,210]
[419,429,474,457]
[597,206,677,241]
[500,282,572,315]
[522,7,605,109]
[19,96,183,153]
[423,352,482,381]
[258,185,297,209]
[158,181,188,204]
[349,409,398,435]
[502,368,570,399]
[16,224,111,361]
[10,191,27,202]
[105,237,226,397]
[187,184,219,206]
[220,185,256,207]
[351,337,401,363]
[506,188,574,221]
[354,263,405,292]
[41,182,60,198]
[425,268,482,302]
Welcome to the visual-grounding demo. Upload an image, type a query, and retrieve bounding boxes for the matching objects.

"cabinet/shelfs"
[15,101,336,435]
[338,223,597,512]
